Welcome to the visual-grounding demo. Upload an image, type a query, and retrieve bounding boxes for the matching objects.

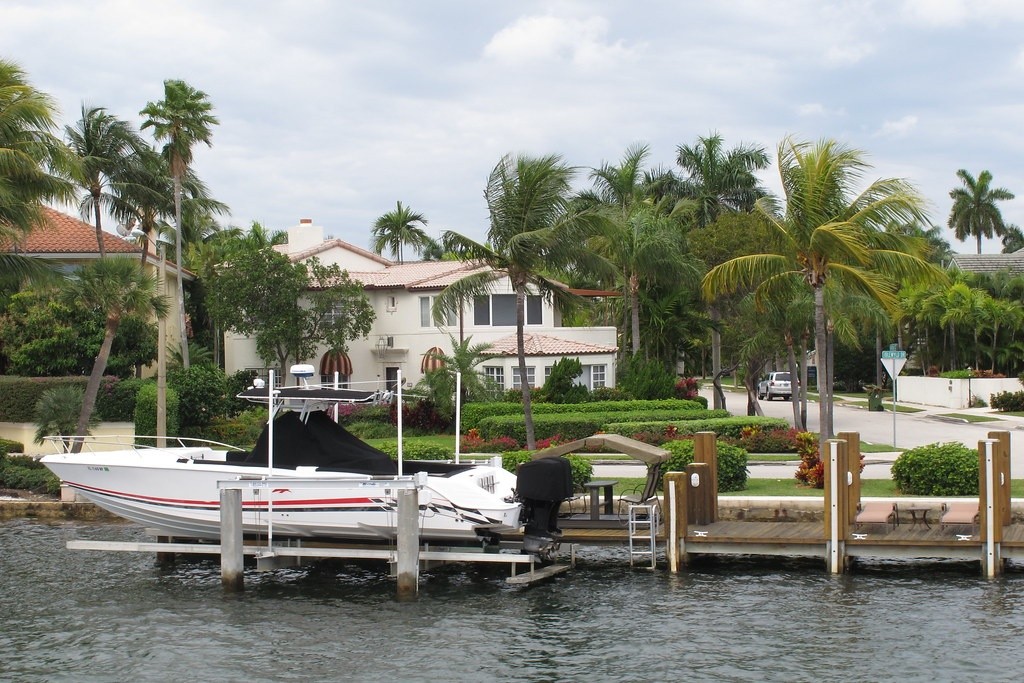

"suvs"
[757,371,795,401]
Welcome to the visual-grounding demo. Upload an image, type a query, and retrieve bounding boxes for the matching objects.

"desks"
[584,481,619,521]
[904,507,932,531]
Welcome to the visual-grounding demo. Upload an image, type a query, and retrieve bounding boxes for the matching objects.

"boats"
[36,363,579,566]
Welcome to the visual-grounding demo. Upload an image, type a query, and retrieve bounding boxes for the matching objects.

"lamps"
[375,333,393,358]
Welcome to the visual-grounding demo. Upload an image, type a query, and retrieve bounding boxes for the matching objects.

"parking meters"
[967,366,973,407]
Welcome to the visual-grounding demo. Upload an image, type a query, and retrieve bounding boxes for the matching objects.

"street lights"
[129,229,169,449]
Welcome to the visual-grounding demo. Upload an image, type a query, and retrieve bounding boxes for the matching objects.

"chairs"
[940,500,980,535]
[560,493,587,520]
[617,462,664,526]
[853,501,899,534]
